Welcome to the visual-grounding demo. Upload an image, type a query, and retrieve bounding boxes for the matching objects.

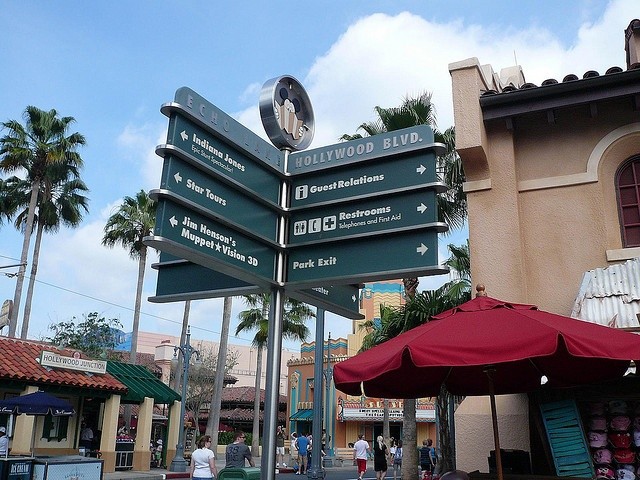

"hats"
[617,469,635,480]
[611,416,630,431]
[157,439,162,444]
[0,426,6,434]
[607,400,627,413]
[590,404,604,415]
[589,417,607,430]
[594,449,612,464]
[596,467,615,479]
[589,432,607,448]
[634,431,640,447]
[613,449,635,463]
[609,433,631,449]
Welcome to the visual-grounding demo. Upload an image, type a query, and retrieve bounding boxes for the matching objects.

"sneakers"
[303,470,307,474]
[283,463,287,468]
[358,477,362,479]
[296,471,300,475]
[276,462,280,468]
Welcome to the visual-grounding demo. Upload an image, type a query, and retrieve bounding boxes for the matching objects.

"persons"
[426,439,437,476]
[276,425,287,467]
[0,426,9,456]
[353,434,371,480]
[225,430,255,468]
[393,439,402,480]
[418,439,434,480]
[374,435,389,480]
[156,439,163,468]
[81,423,94,450]
[189,435,217,480]
[295,428,331,475]
[390,437,396,461]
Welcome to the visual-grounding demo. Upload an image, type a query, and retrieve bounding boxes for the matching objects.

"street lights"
[169,325,203,473]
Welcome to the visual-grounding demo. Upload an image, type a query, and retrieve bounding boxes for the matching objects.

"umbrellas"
[333,282,640,480]
[0,389,77,458]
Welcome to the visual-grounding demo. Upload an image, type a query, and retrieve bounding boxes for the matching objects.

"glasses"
[240,437,245,438]
[208,440,211,443]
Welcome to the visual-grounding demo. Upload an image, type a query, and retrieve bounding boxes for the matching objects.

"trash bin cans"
[217,467,261,480]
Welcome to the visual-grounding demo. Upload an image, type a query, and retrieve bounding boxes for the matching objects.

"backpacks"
[393,448,402,469]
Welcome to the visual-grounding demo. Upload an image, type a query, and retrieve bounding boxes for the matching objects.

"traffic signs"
[289,149,436,208]
[159,152,280,245]
[152,198,276,283]
[165,110,282,206]
[287,227,439,282]
[287,187,438,245]
[301,281,358,314]
